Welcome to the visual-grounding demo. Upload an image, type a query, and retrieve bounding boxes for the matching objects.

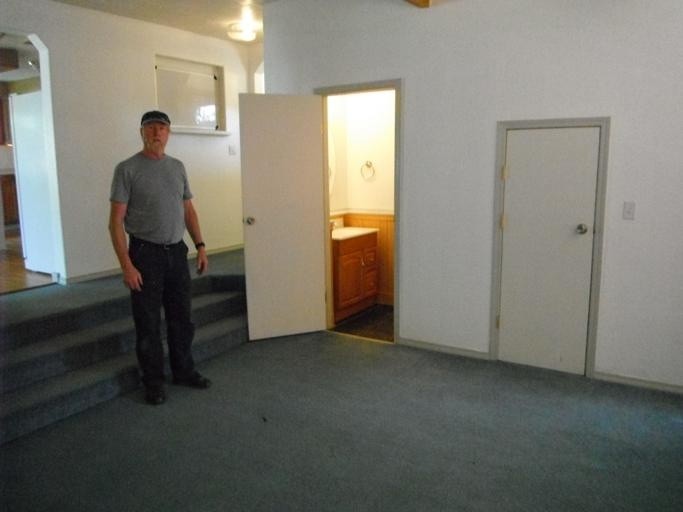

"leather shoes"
[179,373,212,391]
[145,386,167,406]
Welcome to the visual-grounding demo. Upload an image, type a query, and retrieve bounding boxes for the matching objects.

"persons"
[108,109,212,404]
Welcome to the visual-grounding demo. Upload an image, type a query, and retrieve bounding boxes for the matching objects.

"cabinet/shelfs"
[331,233,378,324]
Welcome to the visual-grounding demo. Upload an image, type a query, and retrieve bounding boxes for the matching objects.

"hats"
[138,110,170,128]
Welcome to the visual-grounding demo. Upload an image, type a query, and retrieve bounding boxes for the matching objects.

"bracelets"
[196,243,205,250]
